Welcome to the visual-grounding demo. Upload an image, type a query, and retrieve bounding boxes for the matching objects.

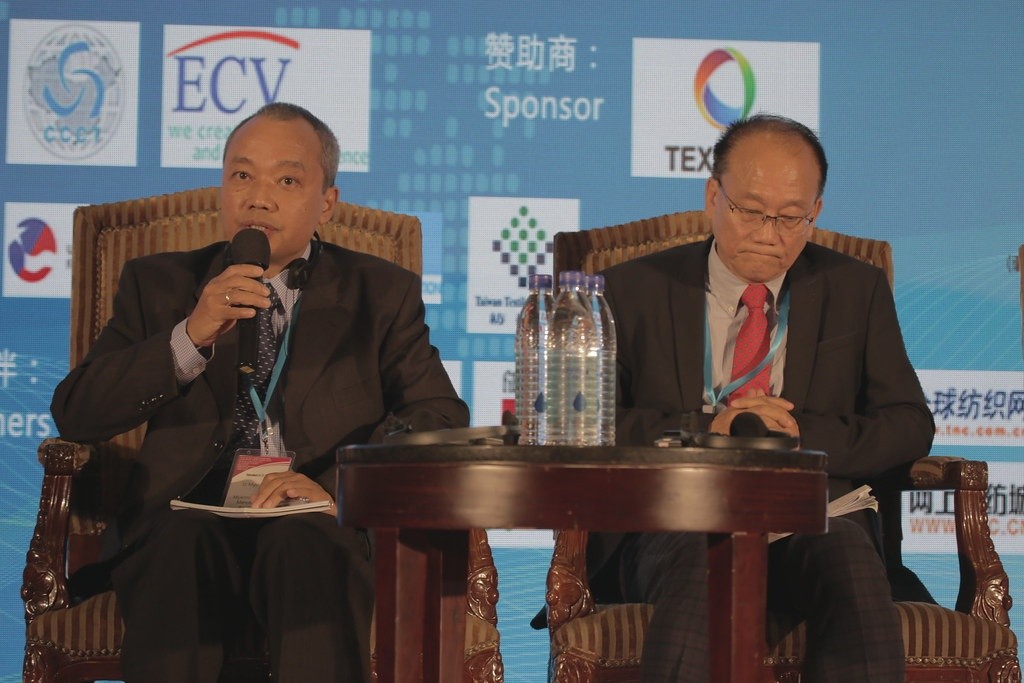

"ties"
[221,283,288,501]
[727,282,771,408]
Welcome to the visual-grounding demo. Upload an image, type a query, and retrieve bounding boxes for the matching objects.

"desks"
[333,446,830,683]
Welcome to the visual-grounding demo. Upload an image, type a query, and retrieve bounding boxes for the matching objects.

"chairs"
[20,186,504,683]
[545,211,1023,683]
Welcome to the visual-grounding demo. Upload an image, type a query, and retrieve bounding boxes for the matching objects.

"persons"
[530,116,937,683]
[50,103,470,683]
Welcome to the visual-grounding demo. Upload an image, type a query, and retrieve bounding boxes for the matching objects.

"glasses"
[716,177,815,229]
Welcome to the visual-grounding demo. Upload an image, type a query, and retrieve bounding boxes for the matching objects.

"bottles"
[584,276,615,448]
[515,274,556,444]
[537,271,599,447]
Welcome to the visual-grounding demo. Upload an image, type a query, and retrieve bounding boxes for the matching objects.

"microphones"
[233,228,271,372]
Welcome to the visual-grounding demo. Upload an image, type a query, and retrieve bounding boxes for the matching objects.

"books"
[170,500,331,518]
[767,485,878,543]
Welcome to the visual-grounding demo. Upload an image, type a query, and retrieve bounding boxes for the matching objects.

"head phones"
[679,411,800,450]
[223,228,321,290]
[381,408,523,445]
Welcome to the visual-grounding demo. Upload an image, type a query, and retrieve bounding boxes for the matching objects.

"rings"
[226,291,231,304]
